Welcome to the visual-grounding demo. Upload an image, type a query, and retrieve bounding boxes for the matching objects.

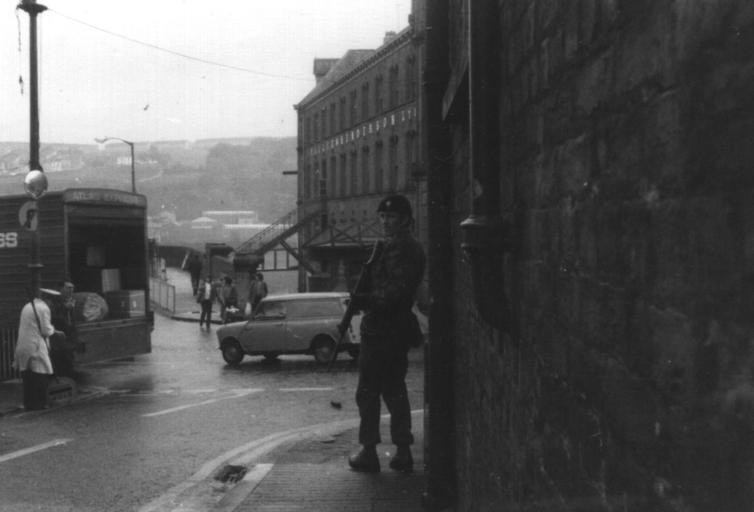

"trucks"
[1,186,156,372]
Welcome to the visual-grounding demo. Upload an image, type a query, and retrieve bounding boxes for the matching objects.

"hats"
[378,196,410,212]
[40,288,61,300]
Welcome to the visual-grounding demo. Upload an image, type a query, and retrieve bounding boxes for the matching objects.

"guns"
[327,239,383,372]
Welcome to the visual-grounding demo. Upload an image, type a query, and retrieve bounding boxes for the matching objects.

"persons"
[341,193,424,474]
[53,283,85,381]
[248,273,267,312]
[216,278,239,324]
[196,275,218,329]
[11,288,64,411]
[188,255,203,296]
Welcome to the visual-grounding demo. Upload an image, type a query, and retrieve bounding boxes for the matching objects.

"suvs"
[215,291,368,367]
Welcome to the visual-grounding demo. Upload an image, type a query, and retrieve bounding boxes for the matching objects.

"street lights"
[95,135,136,194]
